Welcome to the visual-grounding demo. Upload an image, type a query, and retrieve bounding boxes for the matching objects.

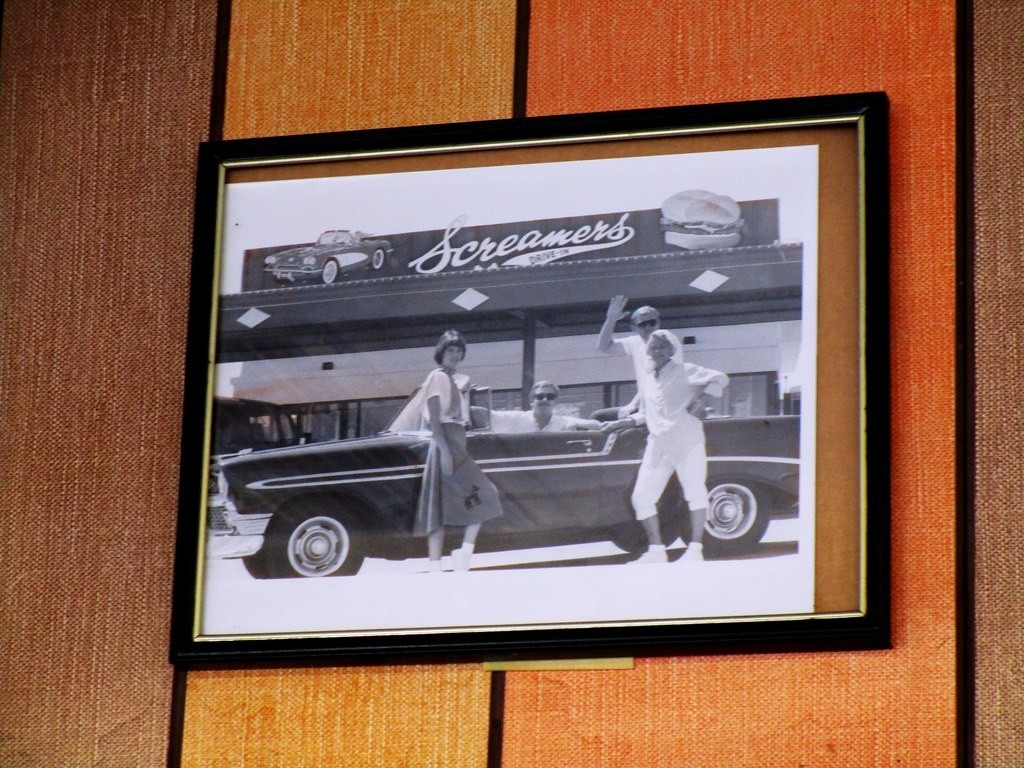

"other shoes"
[631,553,666,567]
[673,550,705,567]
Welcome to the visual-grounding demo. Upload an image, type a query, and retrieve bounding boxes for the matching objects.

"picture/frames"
[165,89,894,669]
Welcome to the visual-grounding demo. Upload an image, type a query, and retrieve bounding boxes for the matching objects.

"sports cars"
[205,381,802,581]
[261,228,395,286]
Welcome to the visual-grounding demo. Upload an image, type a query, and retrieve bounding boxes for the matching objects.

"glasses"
[634,319,658,327]
[535,392,555,401]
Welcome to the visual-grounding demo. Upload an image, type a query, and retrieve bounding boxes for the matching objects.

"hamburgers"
[660,190,745,250]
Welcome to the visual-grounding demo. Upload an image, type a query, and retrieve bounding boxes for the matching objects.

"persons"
[589,296,685,420]
[490,379,601,432]
[415,328,504,572]
[599,329,729,565]
